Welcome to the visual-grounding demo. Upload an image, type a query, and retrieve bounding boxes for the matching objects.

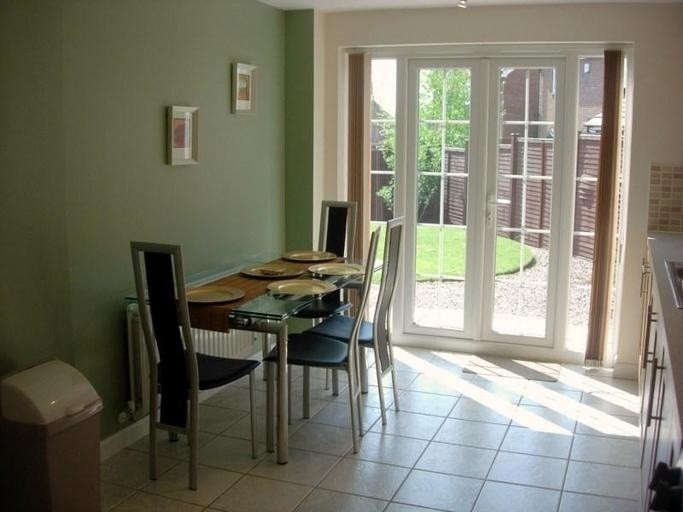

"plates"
[282,250,337,262]
[184,285,247,304]
[264,279,337,296]
[307,263,365,276]
[239,264,304,279]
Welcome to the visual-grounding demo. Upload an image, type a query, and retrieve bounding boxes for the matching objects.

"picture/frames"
[231,61,257,115]
[166,105,200,166]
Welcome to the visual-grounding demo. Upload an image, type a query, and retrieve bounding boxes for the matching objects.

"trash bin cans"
[0,358,105,512]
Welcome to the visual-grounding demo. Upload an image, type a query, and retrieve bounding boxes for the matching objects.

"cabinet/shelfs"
[637,246,681,510]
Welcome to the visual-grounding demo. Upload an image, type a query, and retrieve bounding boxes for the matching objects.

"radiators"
[127,268,256,419]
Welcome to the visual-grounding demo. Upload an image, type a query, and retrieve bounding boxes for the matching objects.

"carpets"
[462,353,562,382]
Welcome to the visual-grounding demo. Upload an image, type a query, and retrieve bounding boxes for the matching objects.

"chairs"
[130,240,260,490]
[302,215,406,426]
[288,200,358,389]
[262,226,381,453]
